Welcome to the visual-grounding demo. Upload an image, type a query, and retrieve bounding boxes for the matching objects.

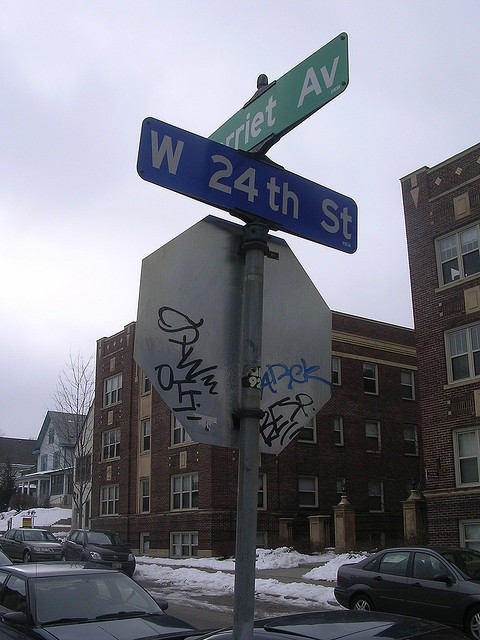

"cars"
[61,528,136,577]
[334,546,480,639]
[0,527,67,563]
[1,560,198,640]
[132,609,479,640]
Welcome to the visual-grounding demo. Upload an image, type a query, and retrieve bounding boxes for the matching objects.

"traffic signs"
[205,32,349,151]
[137,116,357,254]
